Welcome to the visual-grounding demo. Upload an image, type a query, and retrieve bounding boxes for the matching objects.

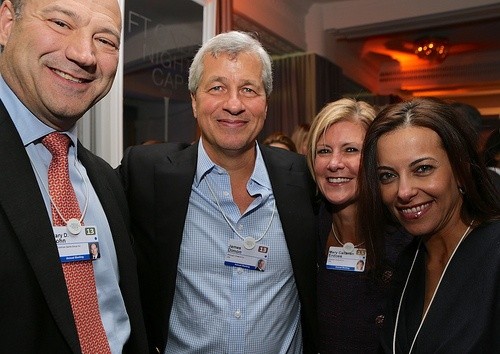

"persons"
[113,32,329,354]
[298,98,415,354]
[0,0,148,354]
[358,98,500,354]
[261,106,500,186]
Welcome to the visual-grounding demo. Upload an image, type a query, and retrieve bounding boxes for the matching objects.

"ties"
[40,132,111,354]
